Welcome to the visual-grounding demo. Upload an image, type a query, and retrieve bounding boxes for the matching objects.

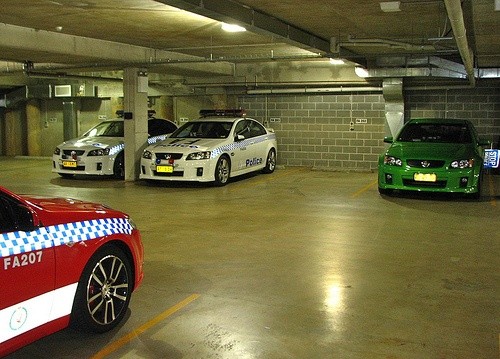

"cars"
[52,110,180,180]
[378,118,490,200]
[0,184,145,359]
[139,109,277,186]
